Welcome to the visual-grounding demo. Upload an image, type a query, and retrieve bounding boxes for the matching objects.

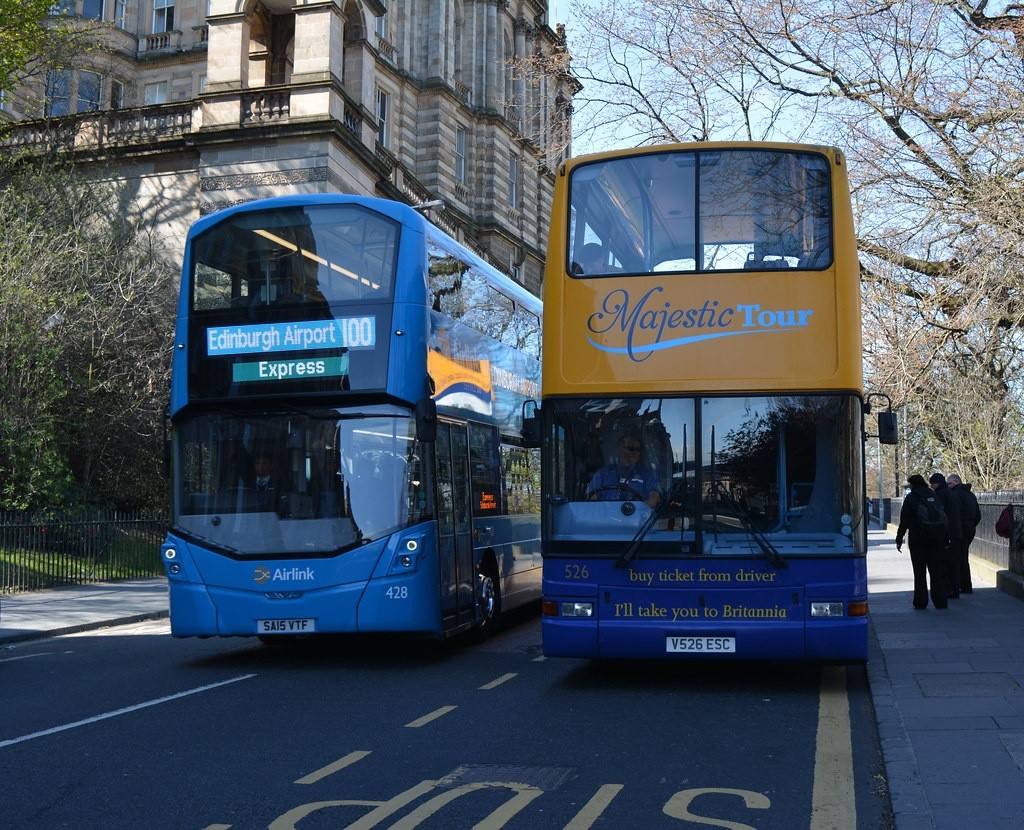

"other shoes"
[947,592,959,599]
[959,589,972,594]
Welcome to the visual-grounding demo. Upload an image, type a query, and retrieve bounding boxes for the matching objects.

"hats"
[930,473,945,483]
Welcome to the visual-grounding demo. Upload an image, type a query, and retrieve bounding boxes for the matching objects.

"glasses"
[621,446,640,452]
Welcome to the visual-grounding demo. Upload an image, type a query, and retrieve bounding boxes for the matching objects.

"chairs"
[744,252,788,269]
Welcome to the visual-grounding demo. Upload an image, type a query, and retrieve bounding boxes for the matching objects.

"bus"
[518,141,900,679]
[161,192,564,642]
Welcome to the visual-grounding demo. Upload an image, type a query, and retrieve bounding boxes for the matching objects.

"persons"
[246,451,290,493]
[583,433,663,507]
[309,450,341,518]
[574,242,625,274]
[894,473,982,610]
[866,497,874,524]
[347,459,382,518]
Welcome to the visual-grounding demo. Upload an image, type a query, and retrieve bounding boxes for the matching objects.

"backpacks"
[908,492,948,542]
